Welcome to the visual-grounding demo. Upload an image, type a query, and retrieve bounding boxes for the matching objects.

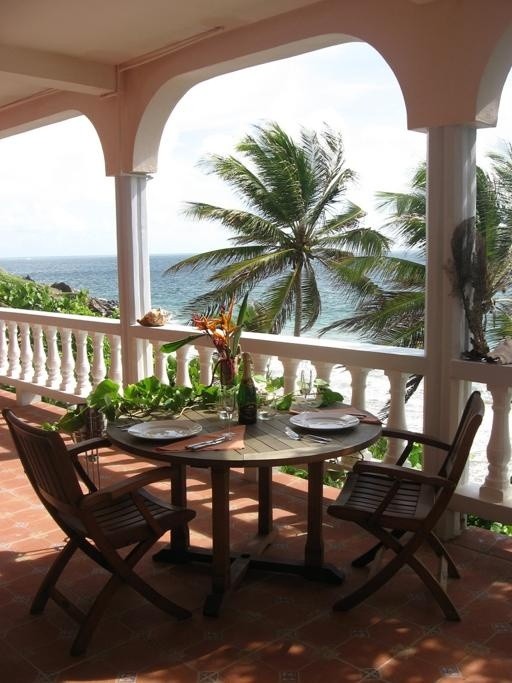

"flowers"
[159,289,249,390]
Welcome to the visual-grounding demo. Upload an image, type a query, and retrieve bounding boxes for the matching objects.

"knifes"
[185,436,225,450]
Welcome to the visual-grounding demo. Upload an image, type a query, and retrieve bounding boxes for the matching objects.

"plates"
[289,412,360,433]
[127,420,202,442]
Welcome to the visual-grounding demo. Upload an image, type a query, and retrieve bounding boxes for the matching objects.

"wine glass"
[299,368,312,403]
[220,385,235,444]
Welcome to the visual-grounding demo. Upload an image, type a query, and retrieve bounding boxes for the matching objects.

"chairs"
[0,405,196,657]
[325,390,486,622]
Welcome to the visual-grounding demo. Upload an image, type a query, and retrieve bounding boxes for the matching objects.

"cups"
[257,394,278,420]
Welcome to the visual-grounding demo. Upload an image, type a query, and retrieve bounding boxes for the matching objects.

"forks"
[284,427,332,445]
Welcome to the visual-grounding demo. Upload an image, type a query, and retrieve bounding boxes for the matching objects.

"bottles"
[237,352,258,425]
